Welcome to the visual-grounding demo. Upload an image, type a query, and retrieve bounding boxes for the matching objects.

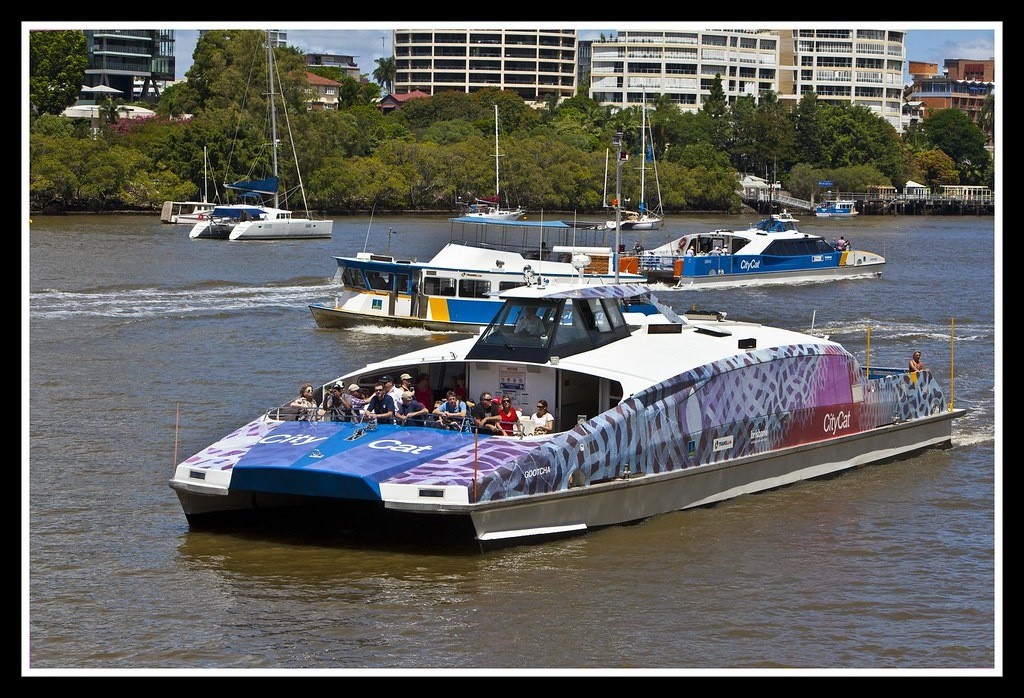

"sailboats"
[463,105,525,220]
[189,30,333,240]
[161,147,221,224]
[607,85,664,231]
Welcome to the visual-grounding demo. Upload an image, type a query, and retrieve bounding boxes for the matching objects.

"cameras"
[329,389,333,392]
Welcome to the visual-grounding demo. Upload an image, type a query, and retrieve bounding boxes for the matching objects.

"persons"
[535,242,550,257]
[909,350,924,372]
[830,236,851,251]
[514,304,546,336]
[708,244,729,256]
[633,240,644,254]
[290,373,554,435]
[687,245,695,257]
[373,272,383,289]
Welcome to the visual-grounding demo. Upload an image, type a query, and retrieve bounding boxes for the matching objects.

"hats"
[348,384,360,391]
[335,381,345,389]
[379,375,393,382]
[400,374,412,380]
[402,392,413,401]
[332,384,341,390]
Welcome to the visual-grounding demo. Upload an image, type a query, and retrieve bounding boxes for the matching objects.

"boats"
[309,205,647,333]
[166,134,966,554]
[635,173,886,285]
[816,198,860,218]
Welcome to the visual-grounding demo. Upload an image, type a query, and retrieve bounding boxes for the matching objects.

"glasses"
[305,390,313,392]
[537,404,544,409]
[484,398,491,401]
[421,377,429,382]
[374,389,382,392]
[502,400,510,403]
